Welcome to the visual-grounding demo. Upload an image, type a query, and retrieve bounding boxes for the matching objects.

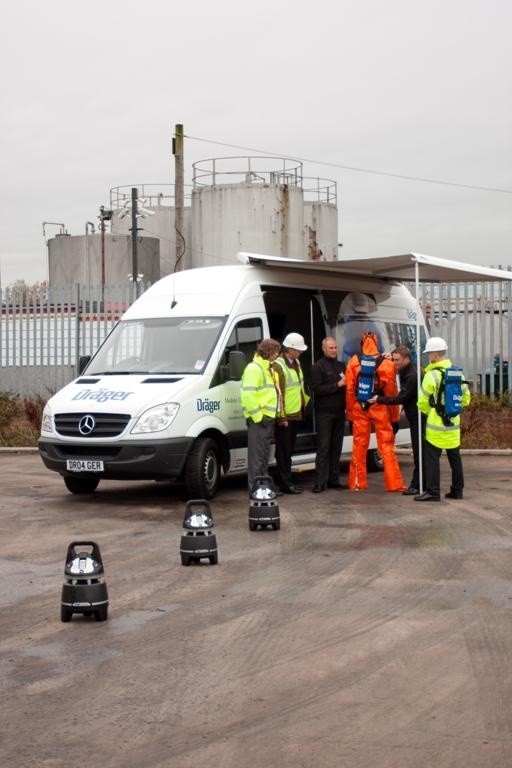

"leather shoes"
[414,493,441,502]
[444,493,463,500]
[402,486,420,495]
[312,482,325,493]
[285,484,304,494]
[327,481,349,490]
[275,490,285,496]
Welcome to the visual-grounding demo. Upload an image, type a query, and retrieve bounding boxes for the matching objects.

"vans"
[39,265,429,500]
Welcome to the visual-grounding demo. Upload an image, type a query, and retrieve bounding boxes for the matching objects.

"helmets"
[422,337,449,353]
[282,332,308,351]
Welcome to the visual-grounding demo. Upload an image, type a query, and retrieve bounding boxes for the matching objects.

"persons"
[413,338,471,500]
[241,338,282,500]
[270,332,307,494]
[309,336,346,493]
[367,347,426,496]
[344,333,407,493]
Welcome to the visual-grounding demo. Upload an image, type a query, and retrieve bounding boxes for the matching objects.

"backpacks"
[355,353,384,410]
[429,367,469,426]
